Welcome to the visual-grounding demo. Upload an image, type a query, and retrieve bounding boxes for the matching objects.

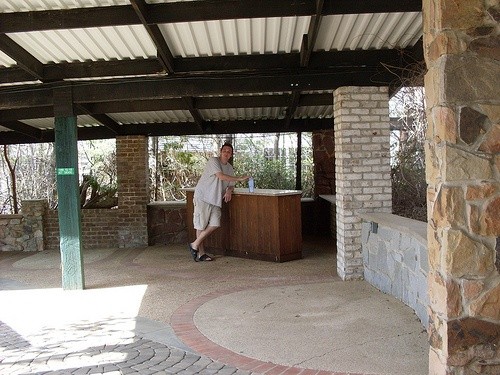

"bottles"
[248,177,254,193]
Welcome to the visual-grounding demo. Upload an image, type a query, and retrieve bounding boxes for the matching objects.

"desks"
[177,188,304,263]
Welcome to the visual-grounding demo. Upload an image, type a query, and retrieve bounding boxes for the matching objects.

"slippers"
[199,254,215,261]
[188,244,198,262]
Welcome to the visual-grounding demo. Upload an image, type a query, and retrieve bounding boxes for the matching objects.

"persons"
[186,143,252,263]
